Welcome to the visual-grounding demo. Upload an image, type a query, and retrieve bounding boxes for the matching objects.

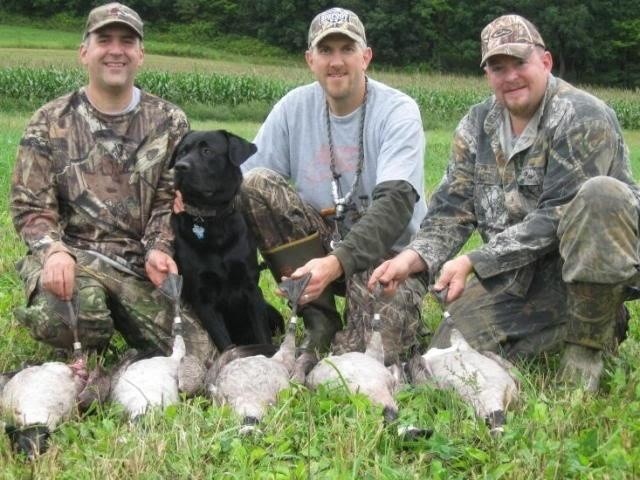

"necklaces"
[313,95,365,249]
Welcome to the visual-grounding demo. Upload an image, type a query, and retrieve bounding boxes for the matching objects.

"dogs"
[167,129,286,357]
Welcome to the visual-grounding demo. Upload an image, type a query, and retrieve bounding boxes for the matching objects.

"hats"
[83,2,146,42]
[479,14,547,67]
[308,5,369,49]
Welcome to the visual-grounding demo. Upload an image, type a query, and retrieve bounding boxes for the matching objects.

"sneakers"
[103,291,148,350]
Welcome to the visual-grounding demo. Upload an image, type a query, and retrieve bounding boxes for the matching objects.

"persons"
[10,1,192,416]
[364,13,640,401]
[170,7,435,391]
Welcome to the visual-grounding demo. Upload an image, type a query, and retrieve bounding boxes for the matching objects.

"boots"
[259,229,344,354]
[546,274,640,402]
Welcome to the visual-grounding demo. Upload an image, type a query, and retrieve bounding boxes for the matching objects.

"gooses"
[306,270,434,445]
[205,270,318,440]
[408,281,522,443]
[109,273,209,422]
[0,298,109,464]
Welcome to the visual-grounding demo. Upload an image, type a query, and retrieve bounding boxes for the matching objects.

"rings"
[304,294,310,304]
